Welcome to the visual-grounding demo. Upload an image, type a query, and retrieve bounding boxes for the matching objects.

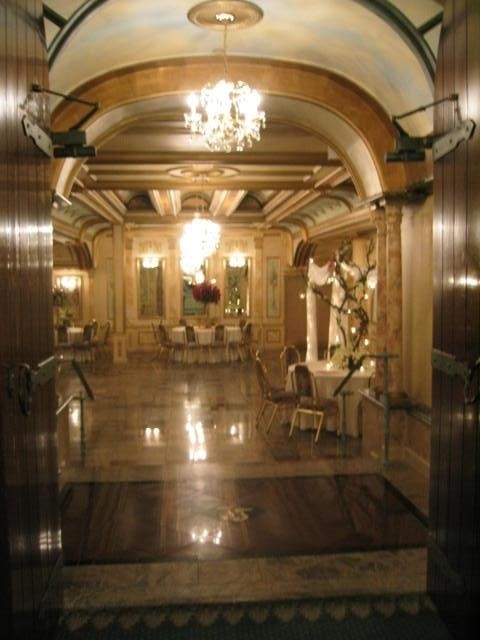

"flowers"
[192,281,222,305]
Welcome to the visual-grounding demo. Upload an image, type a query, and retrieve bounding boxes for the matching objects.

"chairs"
[53,315,112,366]
[252,344,376,443]
[149,318,256,368]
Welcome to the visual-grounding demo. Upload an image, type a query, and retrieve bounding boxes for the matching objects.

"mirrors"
[137,257,162,318]
[223,256,250,317]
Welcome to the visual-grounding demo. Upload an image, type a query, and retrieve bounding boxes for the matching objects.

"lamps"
[180,0,268,153]
